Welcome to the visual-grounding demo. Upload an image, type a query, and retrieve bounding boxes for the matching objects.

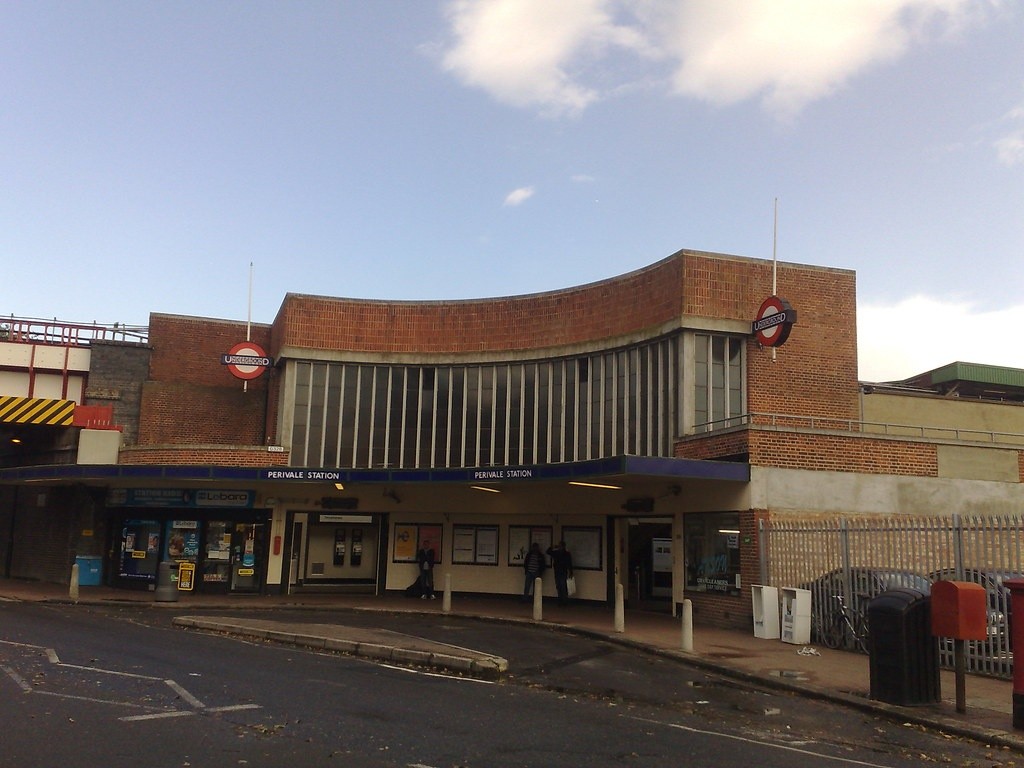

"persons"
[546,541,574,606]
[150,536,159,551]
[125,535,132,548]
[522,542,546,606]
[513,546,524,560]
[420,541,436,599]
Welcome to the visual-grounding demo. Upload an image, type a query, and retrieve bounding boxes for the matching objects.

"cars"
[923,565,1024,629]
[795,564,1008,667]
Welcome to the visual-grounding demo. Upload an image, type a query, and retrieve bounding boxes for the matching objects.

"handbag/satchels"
[567,578,577,596]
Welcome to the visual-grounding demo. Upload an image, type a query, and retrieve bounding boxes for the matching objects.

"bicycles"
[819,595,870,656]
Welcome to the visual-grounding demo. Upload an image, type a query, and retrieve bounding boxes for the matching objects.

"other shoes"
[431,595,436,600]
[421,594,427,600]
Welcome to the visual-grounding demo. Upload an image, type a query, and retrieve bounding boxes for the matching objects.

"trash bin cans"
[153,560,180,601]
[867,587,944,709]
